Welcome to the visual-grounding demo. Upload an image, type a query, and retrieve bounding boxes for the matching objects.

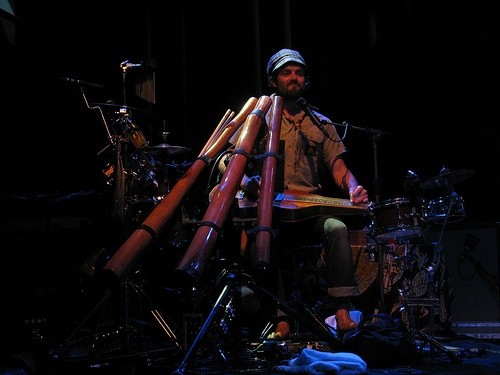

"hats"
[266,48,306,76]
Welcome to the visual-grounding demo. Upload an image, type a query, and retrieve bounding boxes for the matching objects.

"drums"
[115,196,181,243]
[102,150,173,193]
[370,195,422,242]
[424,190,467,226]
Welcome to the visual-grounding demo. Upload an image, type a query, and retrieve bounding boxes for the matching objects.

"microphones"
[64,78,104,89]
[120,60,142,69]
[407,169,420,183]
[297,97,330,138]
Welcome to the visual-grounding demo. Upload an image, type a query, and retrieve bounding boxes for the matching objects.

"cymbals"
[87,98,149,116]
[424,166,475,179]
[142,143,191,154]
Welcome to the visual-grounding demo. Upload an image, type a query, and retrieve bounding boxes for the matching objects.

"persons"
[219,48,383,340]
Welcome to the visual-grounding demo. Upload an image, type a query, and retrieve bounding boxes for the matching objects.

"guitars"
[206,183,388,223]
